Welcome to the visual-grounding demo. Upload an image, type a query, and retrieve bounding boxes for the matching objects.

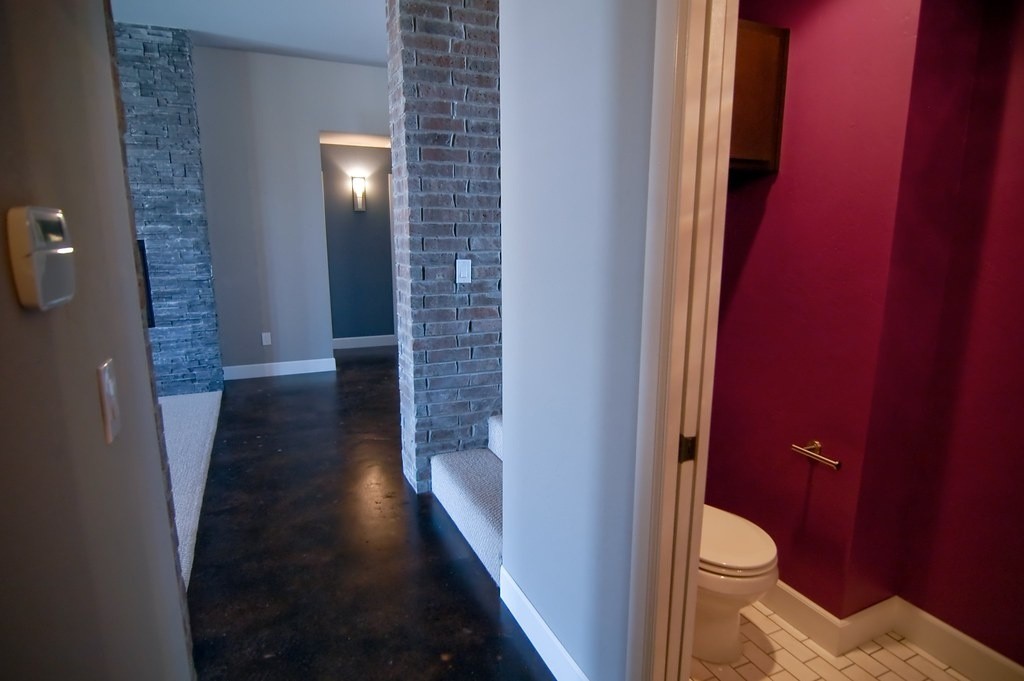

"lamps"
[352,177,367,212]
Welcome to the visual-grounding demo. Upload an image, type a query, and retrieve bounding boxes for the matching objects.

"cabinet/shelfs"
[730,18,786,170]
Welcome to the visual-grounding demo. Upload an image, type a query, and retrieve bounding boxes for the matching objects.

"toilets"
[692,504,779,664]
